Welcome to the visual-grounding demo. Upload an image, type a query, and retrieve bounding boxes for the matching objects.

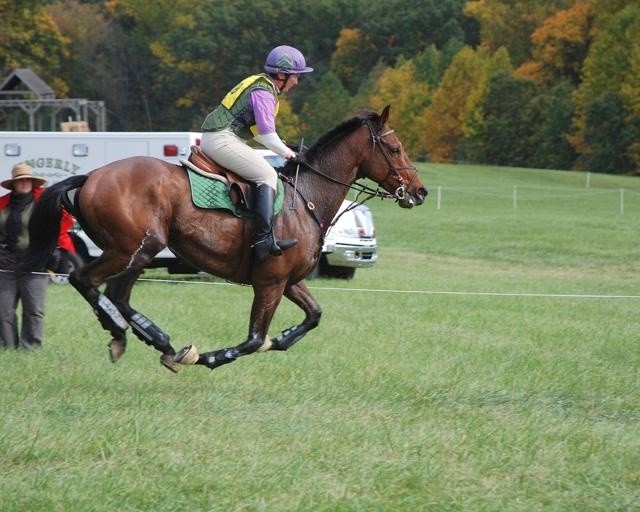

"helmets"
[265,44,314,74]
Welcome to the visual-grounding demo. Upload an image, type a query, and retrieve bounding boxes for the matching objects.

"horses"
[0,105,428,373]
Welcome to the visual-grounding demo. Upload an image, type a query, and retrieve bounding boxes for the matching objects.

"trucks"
[0,129,379,282]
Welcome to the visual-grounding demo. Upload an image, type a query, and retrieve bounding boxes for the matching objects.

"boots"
[253,182,299,265]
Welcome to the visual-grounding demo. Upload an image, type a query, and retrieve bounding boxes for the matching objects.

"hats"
[0,163,46,190]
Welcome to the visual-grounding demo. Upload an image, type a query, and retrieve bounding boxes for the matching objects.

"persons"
[199,45,314,263]
[0,163,77,351]
